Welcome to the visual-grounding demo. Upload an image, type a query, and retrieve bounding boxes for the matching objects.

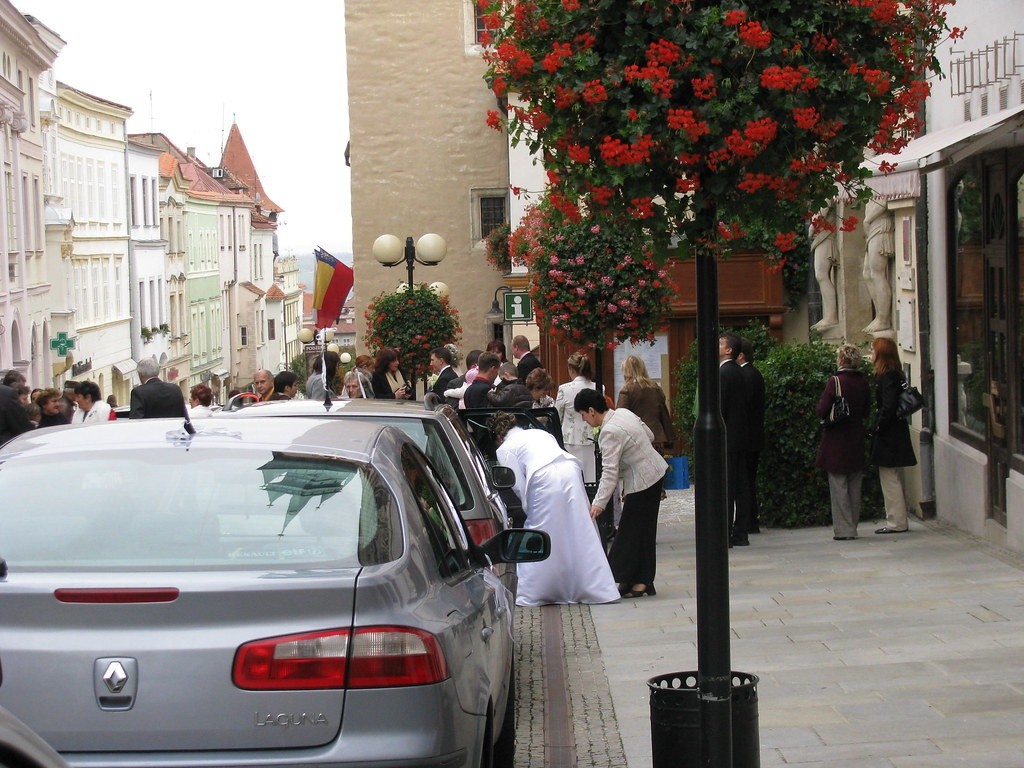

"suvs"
[207,392,567,597]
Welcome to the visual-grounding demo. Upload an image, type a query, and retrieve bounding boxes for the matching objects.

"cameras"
[402,384,412,394]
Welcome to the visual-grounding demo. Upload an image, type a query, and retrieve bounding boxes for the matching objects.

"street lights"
[370,233,445,398]
[396,282,450,393]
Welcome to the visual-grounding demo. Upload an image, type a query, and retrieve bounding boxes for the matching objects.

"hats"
[65,380,79,388]
[465,366,480,385]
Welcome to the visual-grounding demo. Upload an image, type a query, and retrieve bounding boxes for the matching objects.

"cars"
[0,419,552,768]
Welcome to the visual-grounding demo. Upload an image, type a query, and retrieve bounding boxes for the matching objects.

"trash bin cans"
[584,481,614,537]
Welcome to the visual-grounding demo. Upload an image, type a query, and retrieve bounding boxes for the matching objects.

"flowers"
[463,0,971,346]
[363,283,465,385]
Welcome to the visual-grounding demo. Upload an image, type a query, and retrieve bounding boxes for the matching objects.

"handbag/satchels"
[887,370,925,422]
[663,441,690,489]
[819,376,851,427]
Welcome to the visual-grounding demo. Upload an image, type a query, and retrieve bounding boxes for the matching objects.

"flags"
[312,245,354,328]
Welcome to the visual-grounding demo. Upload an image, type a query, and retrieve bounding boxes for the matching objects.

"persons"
[718,334,766,548]
[862,196,895,332]
[227,389,244,410]
[443,335,676,607]
[815,343,871,540]
[809,199,838,330]
[306,344,463,404]
[251,369,298,403]
[128,357,190,422]
[867,337,917,534]
[187,383,213,418]
[0,369,119,448]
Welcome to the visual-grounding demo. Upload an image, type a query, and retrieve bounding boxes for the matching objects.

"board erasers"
[646,671,763,768]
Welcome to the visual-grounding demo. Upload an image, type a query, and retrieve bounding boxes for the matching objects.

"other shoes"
[875,527,909,534]
[833,535,856,540]
[729,527,749,548]
[661,491,668,500]
[749,524,760,533]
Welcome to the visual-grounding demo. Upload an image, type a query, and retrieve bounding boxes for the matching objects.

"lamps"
[486,286,512,326]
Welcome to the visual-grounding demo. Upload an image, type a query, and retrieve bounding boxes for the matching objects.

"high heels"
[618,581,656,598]
